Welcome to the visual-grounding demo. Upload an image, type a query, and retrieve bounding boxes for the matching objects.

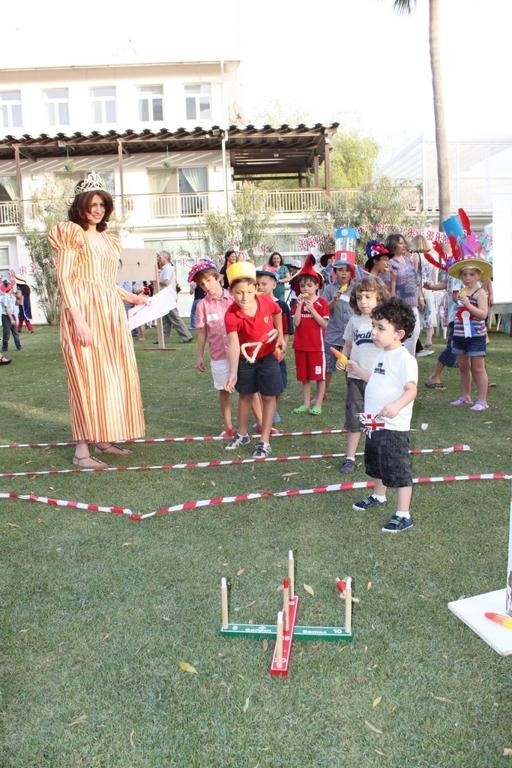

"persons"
[423,275,497,391]
[268,252,293,302]
[1,288,23,351]
[319,254,333,283]
[334,278,392,475]
[452,267,492,412]
[369,255,390,278]
[152,250,194,345]
[15,290,36,333]
[47,170,152,469]
[408,236,438,348]
[353,298,419,533]
[387,235,436,357]
[187,259,280,438]
[0,352,13,366]
[217,250,237,289]
[311,264,361,402]
[190,280,206,330]
[143,281,151,298]
[290,253,330,415]
[222,261,287,458]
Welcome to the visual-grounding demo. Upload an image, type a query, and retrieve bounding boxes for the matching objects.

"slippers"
[424,377,447,392]
[488,380,496,387]
[452,396,474,406]
[470,402,490,412]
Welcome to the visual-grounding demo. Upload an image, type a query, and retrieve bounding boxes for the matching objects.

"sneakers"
[382,514,414,532]
[255,424,279,434]
[352,496,387,510]
[225,433,251,451]
[341,459,357,475]
[417,346,435,356]
[220,429,237,437]
[251,441,272,458]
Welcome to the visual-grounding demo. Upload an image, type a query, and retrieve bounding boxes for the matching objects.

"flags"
[365,416,384,432]
[359,413,379,432]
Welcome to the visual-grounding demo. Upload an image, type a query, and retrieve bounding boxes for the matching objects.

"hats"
[0,278,16,293]
[289,253,322,296]
[333,228,357,280]
[227,262,257,286]
[321,249,335,266]
[448,257,492,282]
[366,240,395,269]
[188,260,216,282]
[256,264,280,283]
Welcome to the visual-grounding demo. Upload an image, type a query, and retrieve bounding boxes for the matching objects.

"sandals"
[0,354,12,365]
[179,337,193,343]
[152,339,166,344]
[310,405,322,416]
[93,445,133,456]
[293,405,311,414]
[72,456,110,469]
